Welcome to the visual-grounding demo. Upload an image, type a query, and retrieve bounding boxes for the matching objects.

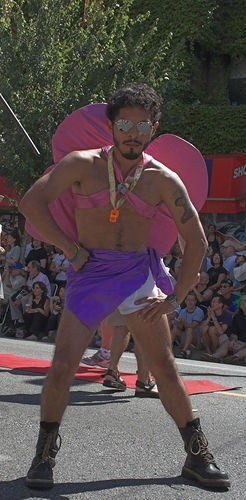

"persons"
[101,308,161,399]
[17,83,233,488]
[0,210,246,381]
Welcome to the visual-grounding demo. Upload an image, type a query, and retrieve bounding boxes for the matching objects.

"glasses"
[113,119,153,135]
[220,285,230,288]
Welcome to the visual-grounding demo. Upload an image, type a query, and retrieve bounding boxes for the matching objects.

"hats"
[220,240,234,247]
[235,250,246,256]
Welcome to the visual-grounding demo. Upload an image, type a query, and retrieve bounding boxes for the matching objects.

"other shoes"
[41,336,48,342]
[224,355,240,364]
[26,336,37,341]
[201,353,221,363]
[175,350,187,358]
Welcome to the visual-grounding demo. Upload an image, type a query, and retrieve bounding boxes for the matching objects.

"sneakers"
[80,349,111,368]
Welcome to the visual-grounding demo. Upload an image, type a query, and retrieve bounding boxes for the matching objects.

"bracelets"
[68,245,80,262]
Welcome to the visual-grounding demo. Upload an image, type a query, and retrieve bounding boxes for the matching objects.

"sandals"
[135,380,159,398]
[100,369,126,390]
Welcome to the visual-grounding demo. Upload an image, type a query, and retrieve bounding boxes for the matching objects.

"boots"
[25,421,61,486]
[178,418,230,487]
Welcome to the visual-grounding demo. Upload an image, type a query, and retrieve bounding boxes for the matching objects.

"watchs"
[165,294,181,311]
